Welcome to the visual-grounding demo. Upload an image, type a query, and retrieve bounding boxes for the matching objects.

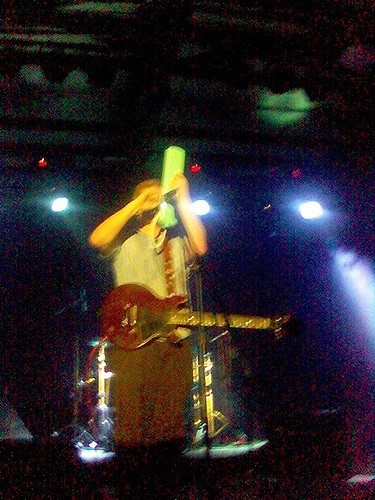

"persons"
[89,174,208,458]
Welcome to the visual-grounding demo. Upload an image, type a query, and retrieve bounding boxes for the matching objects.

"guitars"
[98,283,290,351]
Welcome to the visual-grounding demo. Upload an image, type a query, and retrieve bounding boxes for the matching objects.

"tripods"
[52,342,114,449]
[193,350,248,450]
